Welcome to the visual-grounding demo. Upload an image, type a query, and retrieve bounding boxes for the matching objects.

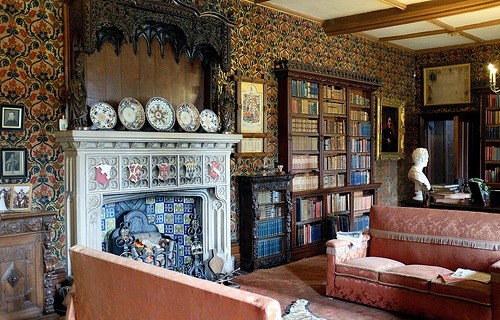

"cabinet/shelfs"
[480,94,500,191]
[239,68,383,274]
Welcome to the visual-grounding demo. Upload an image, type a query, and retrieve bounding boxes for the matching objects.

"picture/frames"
[374,91,407,161]
[419,59,475,108]
[0,147,28,178]
[0,103,25,132]
[0,183,32,212]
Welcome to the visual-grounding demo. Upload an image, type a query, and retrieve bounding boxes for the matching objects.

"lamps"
[487,63,500,94]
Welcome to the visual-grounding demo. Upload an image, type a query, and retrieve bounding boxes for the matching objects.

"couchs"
[325,205,500,320]
[63,244,282,320]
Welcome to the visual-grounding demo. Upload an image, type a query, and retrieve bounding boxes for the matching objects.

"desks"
[398,191,500,213]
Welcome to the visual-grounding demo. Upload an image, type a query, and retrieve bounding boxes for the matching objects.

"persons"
[0,188,29,210]
[4,112,18,126]
[407,147,432,191]
[5,154,18,171]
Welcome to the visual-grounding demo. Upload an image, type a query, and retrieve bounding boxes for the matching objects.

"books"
[481,92,500,183]
[247,78,374,260]
[438,267,491,285]
